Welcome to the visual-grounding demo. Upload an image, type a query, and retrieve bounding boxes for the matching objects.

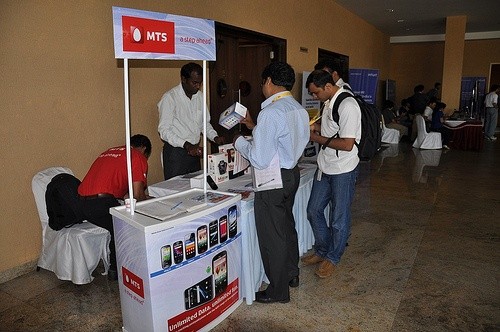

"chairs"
[32,166,111,288]
[411,115,442,150]
[379,114,399,144]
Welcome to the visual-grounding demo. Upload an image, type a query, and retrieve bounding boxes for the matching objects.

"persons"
[384,82,500,145]
[232,60,311,303]
[75,134,152,281]
[301,63,362,279]
[157,63,224,180]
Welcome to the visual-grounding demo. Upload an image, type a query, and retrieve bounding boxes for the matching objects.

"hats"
[416,85,424,90]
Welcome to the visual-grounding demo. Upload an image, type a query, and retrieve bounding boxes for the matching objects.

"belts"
[79,193,113,200]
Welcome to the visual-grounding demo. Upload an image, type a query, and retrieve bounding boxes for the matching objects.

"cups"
[125,198,137,212]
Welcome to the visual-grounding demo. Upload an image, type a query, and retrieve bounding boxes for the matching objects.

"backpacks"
[46,172,84,231]
[320,92,384,162]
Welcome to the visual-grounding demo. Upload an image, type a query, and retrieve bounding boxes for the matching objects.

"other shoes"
[444,142,458,151]
[314,260,337,278]
[400,134,410,144]
[487,135,497,141]
[301,255,324,266]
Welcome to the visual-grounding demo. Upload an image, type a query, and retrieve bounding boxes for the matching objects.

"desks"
[147,157,330,305]
[109,188,243,332]
[442,116,486,152]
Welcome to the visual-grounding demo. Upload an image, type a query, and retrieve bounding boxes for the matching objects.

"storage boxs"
[218,143,244,180]
[219,102,247,129]
[208,153,229,185]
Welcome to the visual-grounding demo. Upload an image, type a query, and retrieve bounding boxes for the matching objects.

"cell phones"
[207,175,219,190]
[161,205,238,310]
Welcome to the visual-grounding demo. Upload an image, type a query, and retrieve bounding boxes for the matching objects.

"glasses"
[145,147,151,157]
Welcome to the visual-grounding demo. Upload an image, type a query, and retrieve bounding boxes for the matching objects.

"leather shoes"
[255,290,290,303]
[289,276,299,287]
